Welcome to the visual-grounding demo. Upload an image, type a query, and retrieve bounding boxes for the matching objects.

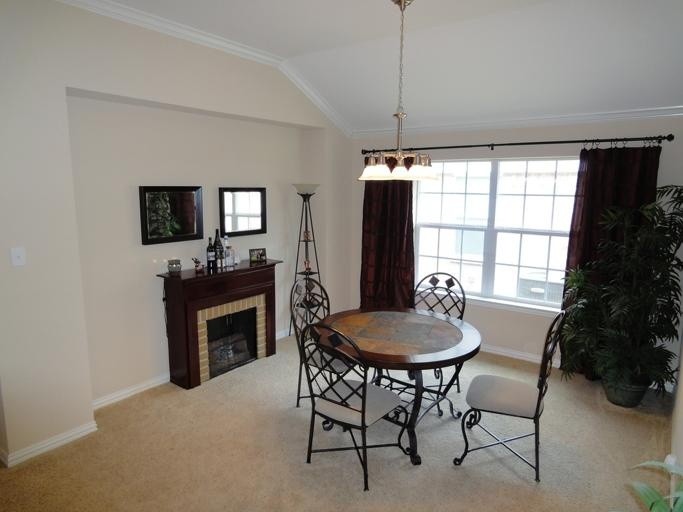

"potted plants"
[560,185,683,407]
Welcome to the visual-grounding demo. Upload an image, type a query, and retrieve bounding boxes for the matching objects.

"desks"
[313,306,481,465]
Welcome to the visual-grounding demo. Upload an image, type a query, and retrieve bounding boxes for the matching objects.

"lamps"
[356,0,439,181]
[291,181,325,322]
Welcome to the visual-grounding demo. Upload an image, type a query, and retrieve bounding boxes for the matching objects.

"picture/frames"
[140,187,204,245]
[249,248,267,267]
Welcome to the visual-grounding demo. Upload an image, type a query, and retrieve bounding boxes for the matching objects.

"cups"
[225,244,234,267]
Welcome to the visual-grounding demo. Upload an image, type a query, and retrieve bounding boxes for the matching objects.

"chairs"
[413,272,466,392]
[300,323,420,490]
[290,277,357,408]
[453,310,568,482]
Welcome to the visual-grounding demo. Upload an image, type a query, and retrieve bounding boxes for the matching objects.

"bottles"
[213,228,224,268]
[168,259,182,276]
[222,234,229,267]
[206,236,216,268]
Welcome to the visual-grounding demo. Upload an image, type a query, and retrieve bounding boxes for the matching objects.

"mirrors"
[218,187,267,238]
